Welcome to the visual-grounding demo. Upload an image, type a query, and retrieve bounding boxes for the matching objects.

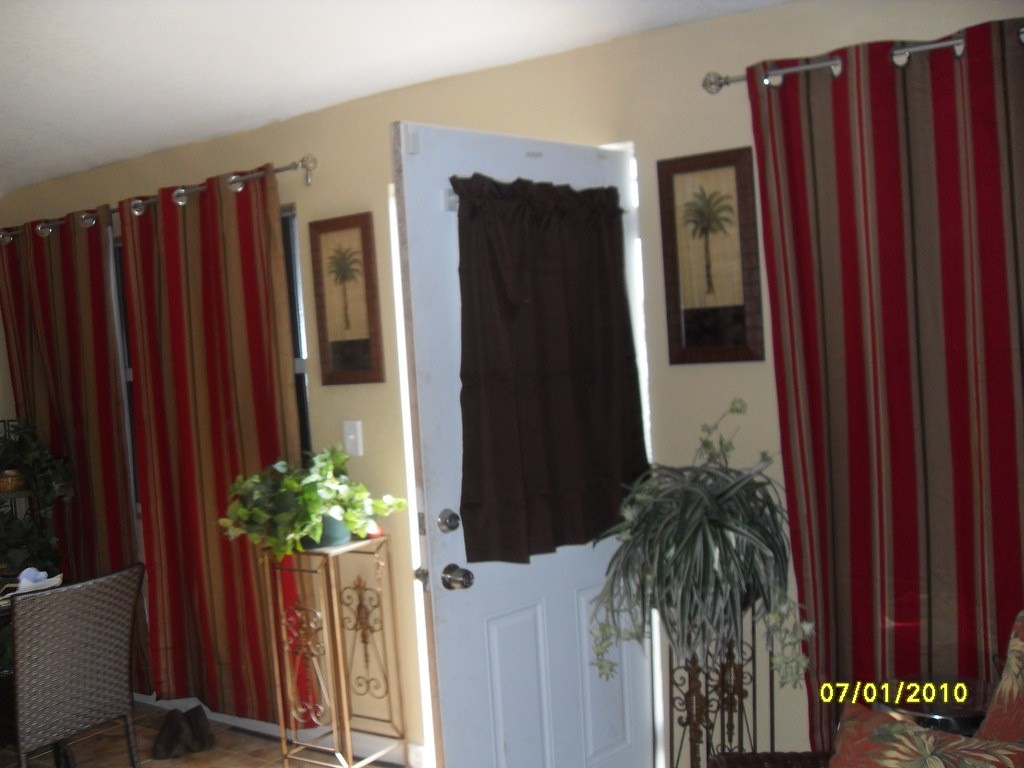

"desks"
[256,534,409,768]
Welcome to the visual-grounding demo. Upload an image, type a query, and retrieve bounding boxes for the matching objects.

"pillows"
[973,611,1023,743]
[829,698,1024,768]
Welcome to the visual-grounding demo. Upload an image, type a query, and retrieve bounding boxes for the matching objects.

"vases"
[301,515,350,549]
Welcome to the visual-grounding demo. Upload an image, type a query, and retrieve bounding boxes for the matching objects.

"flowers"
[220,444,409,563]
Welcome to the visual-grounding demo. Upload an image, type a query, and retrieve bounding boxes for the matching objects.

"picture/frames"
[309,211,385,387]
[657,146,766,365]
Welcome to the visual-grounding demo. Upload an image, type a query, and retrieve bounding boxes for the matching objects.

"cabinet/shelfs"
[0,419,46,543]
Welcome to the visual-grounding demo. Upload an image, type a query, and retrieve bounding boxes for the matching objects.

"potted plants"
[0,413,77,580]
[585,398,815,689]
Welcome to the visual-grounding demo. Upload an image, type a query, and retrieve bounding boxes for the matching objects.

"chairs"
[0,562,144,768]
